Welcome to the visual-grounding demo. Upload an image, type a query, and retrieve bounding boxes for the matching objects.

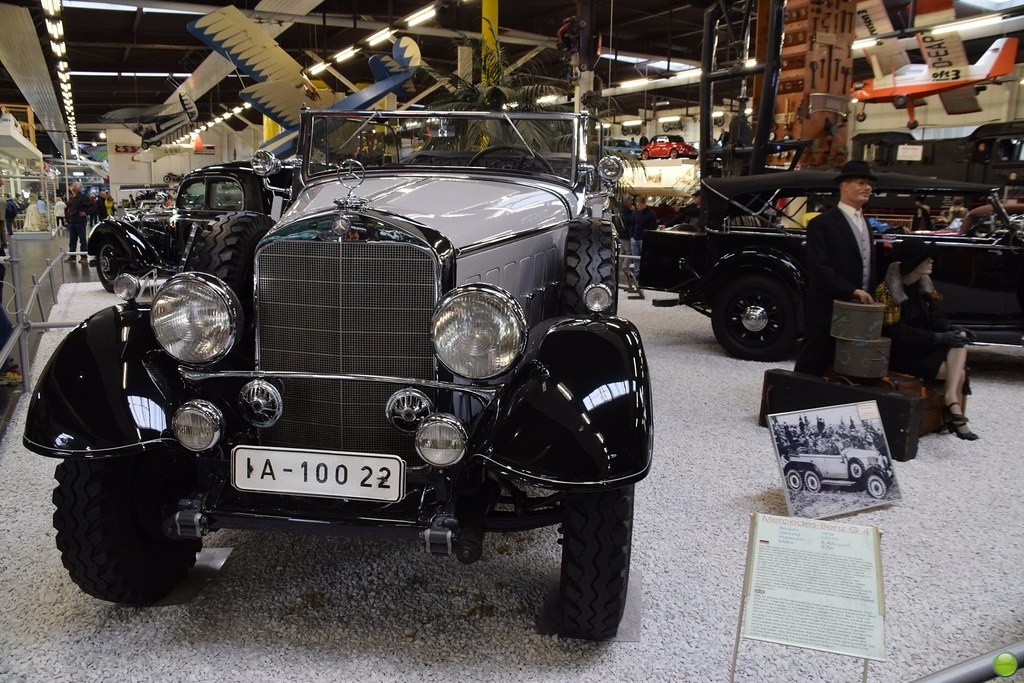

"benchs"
[731,216,762,227]
[412,151,574,175]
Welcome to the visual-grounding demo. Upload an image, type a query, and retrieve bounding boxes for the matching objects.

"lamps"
[306,1,450,77]
[172,101,252,145]
[41,0,78,150]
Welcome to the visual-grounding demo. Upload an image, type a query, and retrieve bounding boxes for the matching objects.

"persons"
[876,239,981,440]
[1,179,118,387]
[619,189,705,286]
[794,160,880,372]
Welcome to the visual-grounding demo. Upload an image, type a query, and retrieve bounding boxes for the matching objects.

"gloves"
[935,329,971,348]
[946,325,977,344]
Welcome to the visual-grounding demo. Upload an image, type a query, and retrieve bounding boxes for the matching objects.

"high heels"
[949,421,979,441]
[944,402,969,422]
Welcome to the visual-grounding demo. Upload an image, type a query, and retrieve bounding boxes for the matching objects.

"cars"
[20,98,1023,641]
[781,441,892,502]
[604,139,643,160]
[641,134,698,160]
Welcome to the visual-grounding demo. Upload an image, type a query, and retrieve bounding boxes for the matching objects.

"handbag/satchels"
[833,336,891,377]
[830,296,886,341]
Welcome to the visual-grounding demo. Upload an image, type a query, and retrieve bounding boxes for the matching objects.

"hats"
[692,189,702,197]
[898,236,937,277]
[832,159,878,185]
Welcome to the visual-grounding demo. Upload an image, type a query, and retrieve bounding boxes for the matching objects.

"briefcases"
[758,356,947,462]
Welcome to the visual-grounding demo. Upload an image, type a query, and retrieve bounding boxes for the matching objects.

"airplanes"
[130,132,203,163]
[99,93,198,149]
[846,0,1020,131]
[186,4,422,163]
